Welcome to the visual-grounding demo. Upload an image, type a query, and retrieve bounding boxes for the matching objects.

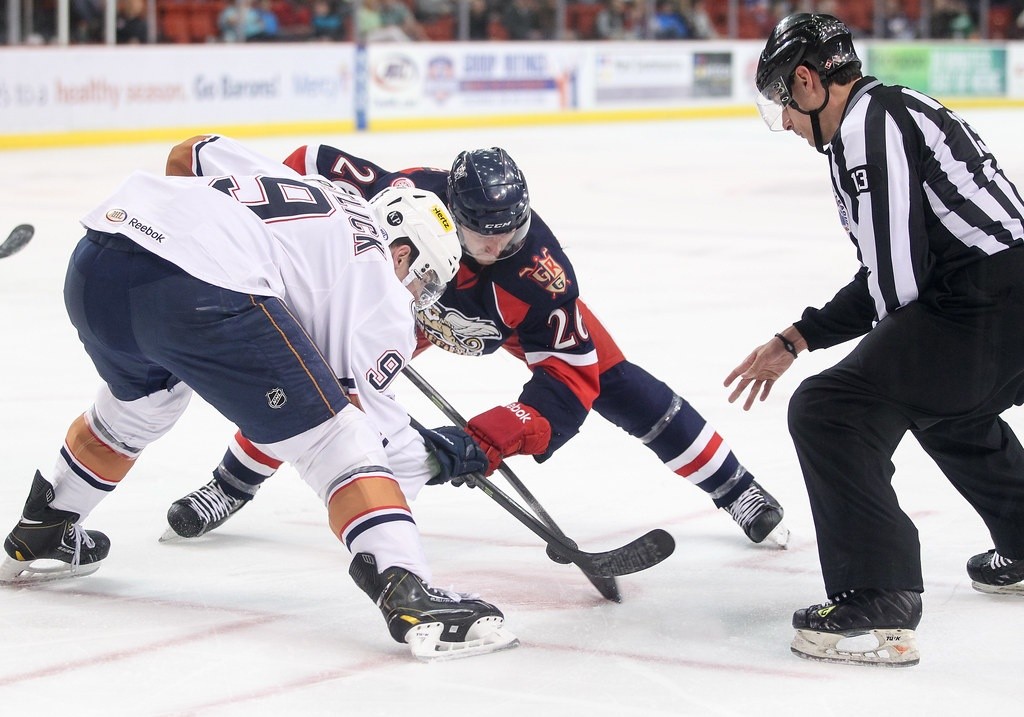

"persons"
[0,135,521,663]
[0,0,1024,46]
[722,11,1024,666]
[160,147,789,550]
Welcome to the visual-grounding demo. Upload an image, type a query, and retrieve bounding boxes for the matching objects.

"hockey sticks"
[0,223,37,262]
[401,363,626,606]
[389,392,679,581]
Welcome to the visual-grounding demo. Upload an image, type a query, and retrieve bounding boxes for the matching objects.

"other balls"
[545,536,579,565]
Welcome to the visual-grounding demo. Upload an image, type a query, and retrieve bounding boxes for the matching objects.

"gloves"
[464,402,550,478]
[414,425,488,488]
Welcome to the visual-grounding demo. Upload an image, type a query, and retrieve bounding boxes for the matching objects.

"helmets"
[446,147,531,261]
[756,12,862,132]
[368,186,462,310]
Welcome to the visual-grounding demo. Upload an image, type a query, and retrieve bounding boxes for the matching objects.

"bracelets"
[774,333,798,359]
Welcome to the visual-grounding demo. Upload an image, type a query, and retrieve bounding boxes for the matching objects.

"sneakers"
[0,469,110,586]
[349,553,521,662]
[791,590,922,667]
[158,478,249,543]
[721,480,790,550]
[967,548,1024,596]
